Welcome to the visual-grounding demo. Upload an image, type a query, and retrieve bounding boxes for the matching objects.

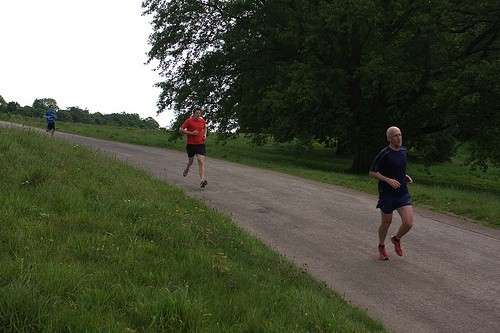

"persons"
[44,104,57,135]
[368,125,414,260]
[180,107,208,188]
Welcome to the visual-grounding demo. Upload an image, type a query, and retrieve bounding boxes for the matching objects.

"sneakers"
[378,244,389,260]
[200,178,208,189]
[391,235,403,256]
[183,167,189,176]
